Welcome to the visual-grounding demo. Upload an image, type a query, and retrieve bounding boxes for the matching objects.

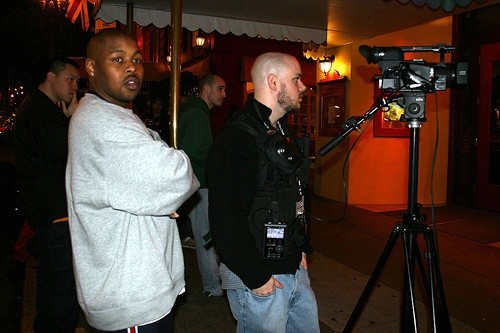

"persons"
[16,55,85,333]
[207,51,320,333]
[64,28,201,333]
[148,74,228,301]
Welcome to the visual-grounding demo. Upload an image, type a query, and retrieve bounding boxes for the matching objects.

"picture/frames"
[373,74,419,138]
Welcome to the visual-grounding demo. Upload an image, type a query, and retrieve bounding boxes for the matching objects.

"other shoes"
[207,283,225,297]
[181,237,196,249]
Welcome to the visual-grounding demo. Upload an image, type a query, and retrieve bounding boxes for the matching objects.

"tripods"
[319,88,452,333]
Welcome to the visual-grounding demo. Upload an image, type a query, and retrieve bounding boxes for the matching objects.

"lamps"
[318,55,335,76]
[196,28,205,47]
